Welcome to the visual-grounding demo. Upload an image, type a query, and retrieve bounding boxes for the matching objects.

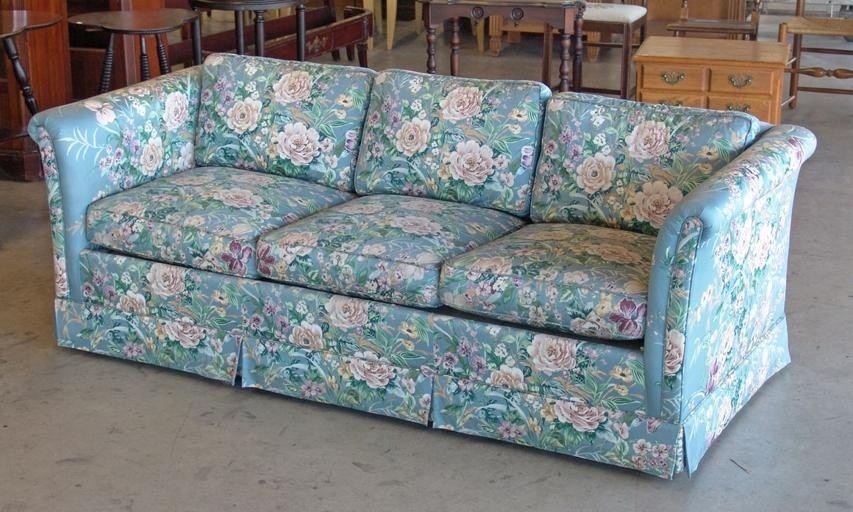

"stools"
[68,8,199,95]
[542,2,647,99]
[186,0,311,65]
[0,9,63,178]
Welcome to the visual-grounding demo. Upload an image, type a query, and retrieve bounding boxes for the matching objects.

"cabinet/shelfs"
[631,35,790,126]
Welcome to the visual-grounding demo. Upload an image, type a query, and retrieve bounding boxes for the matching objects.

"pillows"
[530,92,760,237]
[354,67,553,216]
[195,52,378,196]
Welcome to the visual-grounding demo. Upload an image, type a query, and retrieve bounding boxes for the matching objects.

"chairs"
[778,0,853,109]
[666,0,763,41]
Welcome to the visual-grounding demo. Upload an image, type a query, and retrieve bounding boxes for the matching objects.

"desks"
[416,0,585,92]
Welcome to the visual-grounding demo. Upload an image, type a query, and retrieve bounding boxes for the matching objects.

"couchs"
[27,52,818,482]
